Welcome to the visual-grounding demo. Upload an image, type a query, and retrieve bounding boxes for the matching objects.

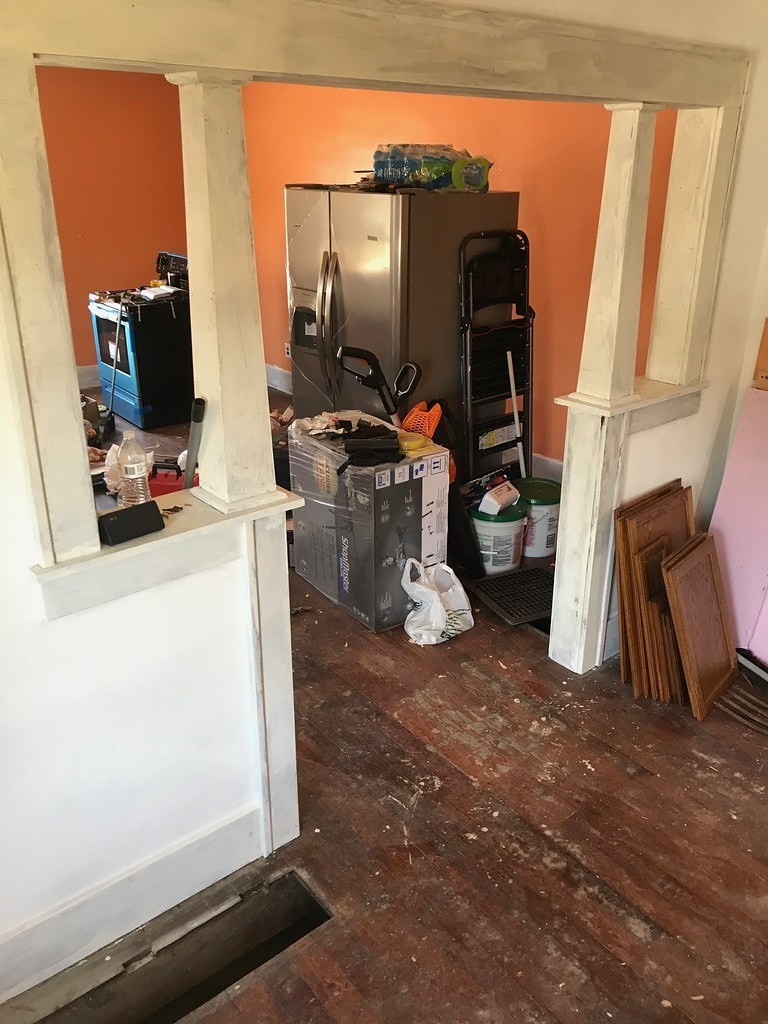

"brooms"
[104,301,123,432]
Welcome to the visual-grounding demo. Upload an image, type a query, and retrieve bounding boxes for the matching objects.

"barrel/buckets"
[466,496,527,576]
[510,477,561,558]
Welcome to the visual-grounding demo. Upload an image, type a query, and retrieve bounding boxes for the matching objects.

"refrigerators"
[282,182,521,518]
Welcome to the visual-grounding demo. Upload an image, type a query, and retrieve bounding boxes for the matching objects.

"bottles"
[373,143,453,191]
[116,430,151,505]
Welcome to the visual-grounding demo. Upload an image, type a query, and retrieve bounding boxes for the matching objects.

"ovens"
[88,285,195,429]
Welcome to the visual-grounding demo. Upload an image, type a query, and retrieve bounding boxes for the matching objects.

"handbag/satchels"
[401,558,474,645]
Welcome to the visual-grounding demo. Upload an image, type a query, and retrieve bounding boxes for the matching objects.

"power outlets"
[285,342,291,358]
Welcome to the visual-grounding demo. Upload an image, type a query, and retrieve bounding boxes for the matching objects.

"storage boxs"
[287,410,450,634]
[81,394,101,435]
